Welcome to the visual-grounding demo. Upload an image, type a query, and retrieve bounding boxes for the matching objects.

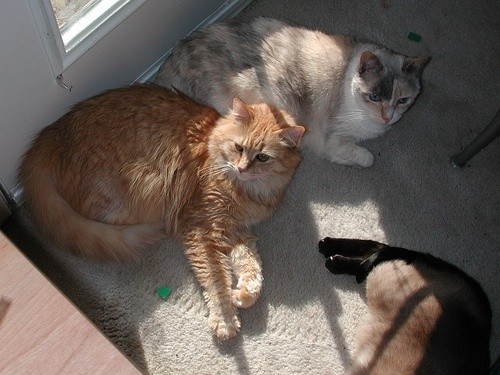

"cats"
[316,237,493,375]
[14,82,306,341]
[153,14,434,171]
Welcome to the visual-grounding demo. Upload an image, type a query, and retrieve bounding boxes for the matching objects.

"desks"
[0,231,146,375]
